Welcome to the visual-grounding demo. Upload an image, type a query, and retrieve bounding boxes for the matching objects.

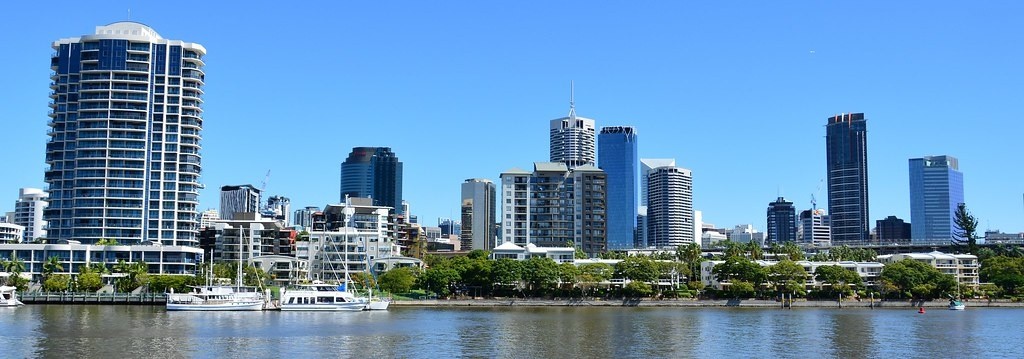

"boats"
[0,284,20,308]
[947,300,966,311]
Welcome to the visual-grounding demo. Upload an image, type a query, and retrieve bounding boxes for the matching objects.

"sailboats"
[164,225,271,313]
[277,191,395,311]
